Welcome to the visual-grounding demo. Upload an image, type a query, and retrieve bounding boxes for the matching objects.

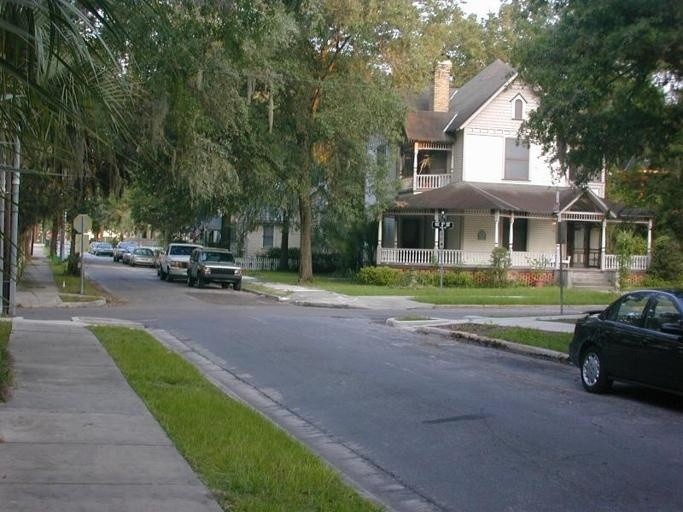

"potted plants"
[524,254,555,290]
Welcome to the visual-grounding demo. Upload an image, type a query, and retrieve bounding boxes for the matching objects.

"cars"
[88,241,241,291]
[569,288,682,399]
